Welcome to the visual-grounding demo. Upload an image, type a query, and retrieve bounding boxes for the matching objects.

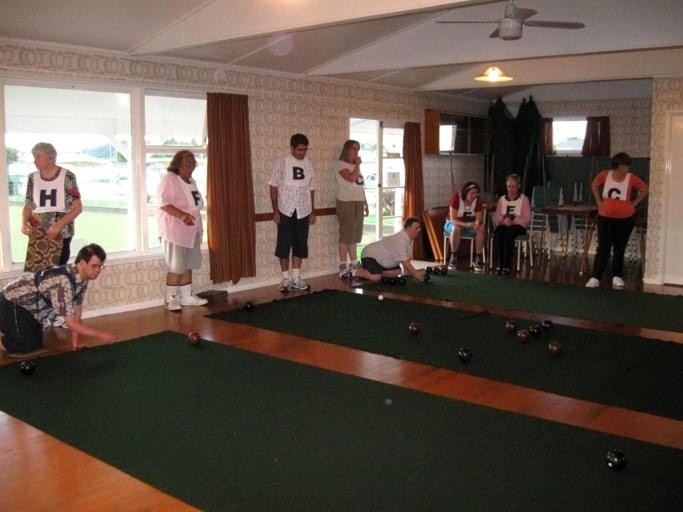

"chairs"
[440,178,648,272]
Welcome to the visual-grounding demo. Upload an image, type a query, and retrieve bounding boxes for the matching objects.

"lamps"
[474,65,514,85]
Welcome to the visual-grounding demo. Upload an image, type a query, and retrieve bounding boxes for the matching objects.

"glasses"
[88,264,104,271]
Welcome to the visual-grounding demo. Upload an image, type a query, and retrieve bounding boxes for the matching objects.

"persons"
[337,217,429,284]
[1,244,119,353]
[443,181,487,272]
[266,133,316,292]
[21,142,84,327]
[493,173,532,279]
[158,148,208,312]
[585,152,649,289]
[334,140,368,271]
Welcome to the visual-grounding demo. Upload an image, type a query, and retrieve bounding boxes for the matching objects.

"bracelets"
[180,214,190,223]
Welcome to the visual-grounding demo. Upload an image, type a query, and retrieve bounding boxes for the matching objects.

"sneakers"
[472,257,482,272]
[181,291,207,305]
[291,279,309,290]
[448,258,456,270]
[163,295,181,311]
[502,270,511,278]
[53,316,66,328]
[585,277,599,287]
[338,265,346,278]
[495,268,502,277]
[279,278,290,292]
[339,267,354,278]
[612,276,624,286]
[349,263,356,272]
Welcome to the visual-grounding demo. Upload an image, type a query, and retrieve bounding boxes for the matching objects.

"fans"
[433,0,585,42]
